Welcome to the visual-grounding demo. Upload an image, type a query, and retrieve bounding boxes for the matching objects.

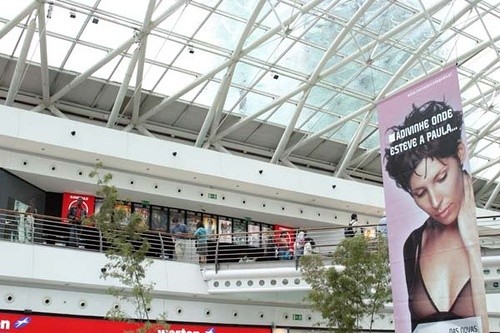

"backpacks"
[344,221,356,238]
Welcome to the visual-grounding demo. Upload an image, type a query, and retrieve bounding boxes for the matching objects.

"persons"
[194,222,208,264]
[382,98,490,333]
[9,196,37,243]
[170,217,189,262]
[275,211,388,259]
[66,198,87,249]
